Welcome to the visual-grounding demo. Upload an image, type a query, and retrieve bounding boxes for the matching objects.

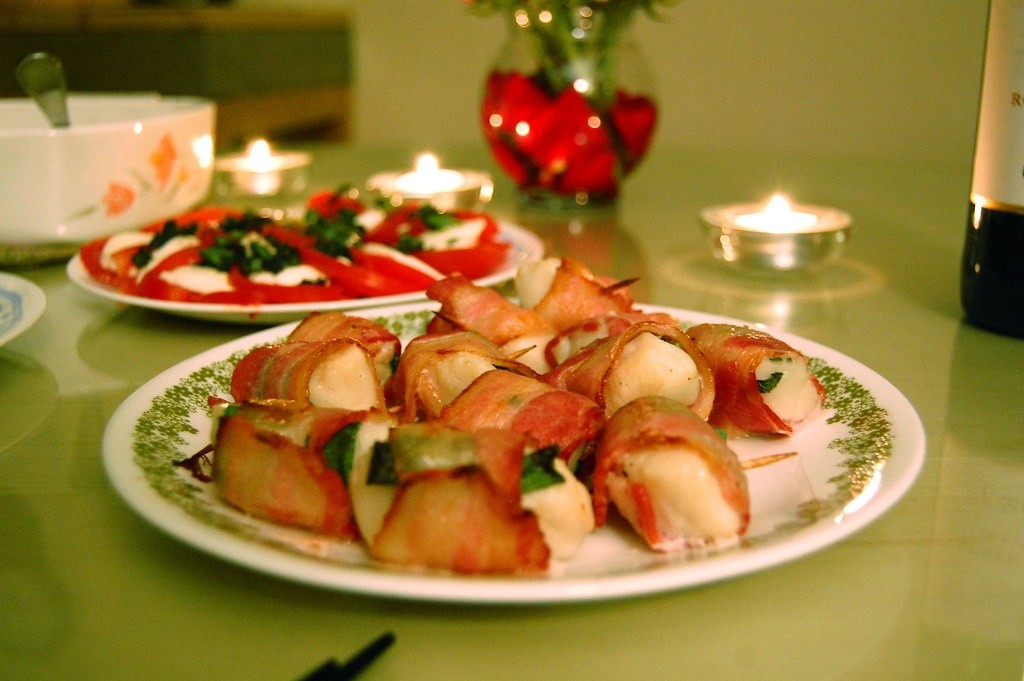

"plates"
[102,298,926,605]
[0,272,47,346]
[65,215,546,321]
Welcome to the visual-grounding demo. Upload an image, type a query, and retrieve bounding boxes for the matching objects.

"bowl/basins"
[0,95,217,265]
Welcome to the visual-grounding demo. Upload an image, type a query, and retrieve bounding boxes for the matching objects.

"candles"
[394,152,464,195]
[736,188,817,233]
[235,137,283,171]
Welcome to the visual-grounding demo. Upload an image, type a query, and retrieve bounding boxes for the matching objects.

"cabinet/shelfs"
[0,6,356,156]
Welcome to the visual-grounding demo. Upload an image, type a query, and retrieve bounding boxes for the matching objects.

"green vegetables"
[133,194,459,276]
[320,421,588,494]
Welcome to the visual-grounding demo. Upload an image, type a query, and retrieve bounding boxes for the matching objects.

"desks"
[1,144,1024,681]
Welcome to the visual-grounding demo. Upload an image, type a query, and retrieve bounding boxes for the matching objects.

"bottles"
[960,0,1023,340]
